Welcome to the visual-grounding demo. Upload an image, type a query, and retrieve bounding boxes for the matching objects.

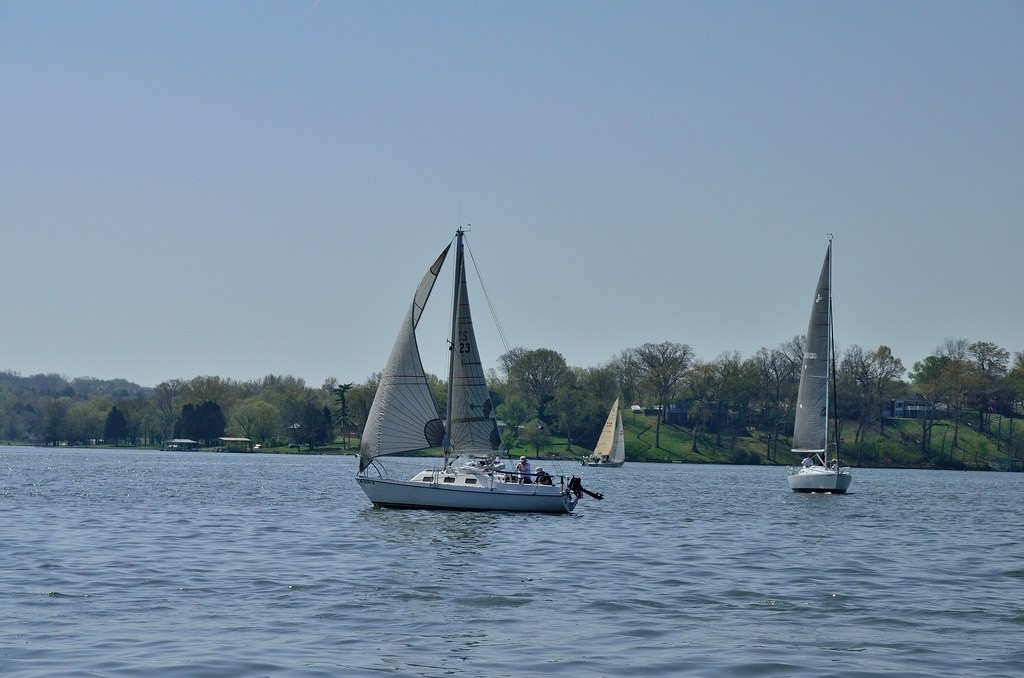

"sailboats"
[579,393,627,467]
[351,223,606,514]
[785,233,853,494]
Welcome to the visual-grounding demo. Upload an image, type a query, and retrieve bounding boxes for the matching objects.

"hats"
[832,458,837,461]
[534,467,544,473]
[519,456,527,462]
[496,454,499,456]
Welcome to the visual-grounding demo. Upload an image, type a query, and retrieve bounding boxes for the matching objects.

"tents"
[166,438,197,444]
[210,436,251,444]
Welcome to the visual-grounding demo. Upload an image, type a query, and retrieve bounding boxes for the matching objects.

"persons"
[516,455,532,484]
[817,451,824,465]
[831,458,837,468]
[534,467,552,485]
[582,455,590,465]
[801,454,815,468]
[488,454,500,465]
[593,454,607,463]
